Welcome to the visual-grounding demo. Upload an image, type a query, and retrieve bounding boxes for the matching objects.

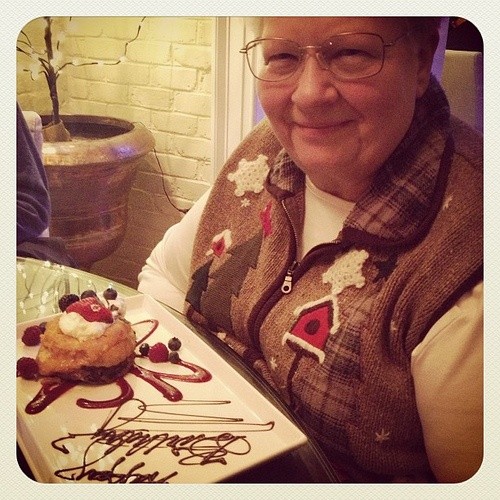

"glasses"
[238,26,406,84]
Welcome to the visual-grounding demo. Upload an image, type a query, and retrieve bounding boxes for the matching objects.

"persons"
[137,15,484,485]
[17,102,76,267]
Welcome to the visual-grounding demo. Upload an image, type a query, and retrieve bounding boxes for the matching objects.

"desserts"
[34,288,137,385]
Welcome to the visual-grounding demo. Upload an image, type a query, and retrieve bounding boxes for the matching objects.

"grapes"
[16,357,38,380]
[21,326,42,346]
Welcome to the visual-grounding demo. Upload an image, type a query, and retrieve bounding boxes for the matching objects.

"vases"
[39,114,154,272]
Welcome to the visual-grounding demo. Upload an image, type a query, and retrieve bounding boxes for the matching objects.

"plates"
[16,295,308,483]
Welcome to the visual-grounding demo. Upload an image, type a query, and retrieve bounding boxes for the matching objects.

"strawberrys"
[148,343,169,363]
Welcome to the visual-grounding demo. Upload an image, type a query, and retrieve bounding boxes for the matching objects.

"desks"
[17,256,340,484]
